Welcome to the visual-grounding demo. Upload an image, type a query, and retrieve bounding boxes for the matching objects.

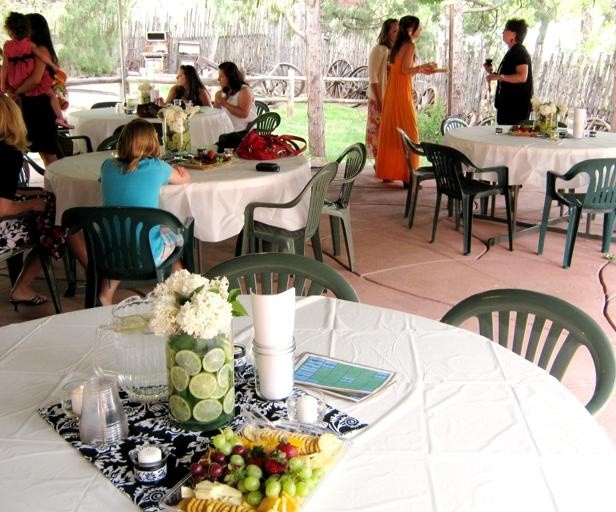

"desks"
[44,143,313,273]
[440,125,616,240]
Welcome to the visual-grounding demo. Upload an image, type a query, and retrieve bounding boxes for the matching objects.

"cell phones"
[496,128,502,133]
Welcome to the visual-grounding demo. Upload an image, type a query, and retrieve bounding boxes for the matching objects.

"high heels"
[9,295,47,311]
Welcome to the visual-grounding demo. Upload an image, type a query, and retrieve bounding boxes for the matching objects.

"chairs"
[0,189,72,314]
[3,100,281,189]
[421,141,522,255]
[75,203,196,308]
[438,287,616,415]
[200,251,359,305]
[441,115,612,136]
[395,127,467,230]
[309,142,368,273]
[241,161,338,262]
[537,159,614,269]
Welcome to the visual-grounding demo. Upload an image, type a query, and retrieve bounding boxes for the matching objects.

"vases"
[166,335,236,430]
[138,85,151,104]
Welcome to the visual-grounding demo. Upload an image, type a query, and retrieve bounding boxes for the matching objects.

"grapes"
[188,152,231,168]
[191,428,324,507]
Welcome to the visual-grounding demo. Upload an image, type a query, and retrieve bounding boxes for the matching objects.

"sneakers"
[55,118,73,128]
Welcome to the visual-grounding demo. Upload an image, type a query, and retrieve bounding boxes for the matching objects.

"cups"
[95,292,179,403]
[166,145,236,169]
[485,53,496,69]
[128,442,171,486]
[57,376,136,446]
[511,102,597,141]
[251,338,298,404]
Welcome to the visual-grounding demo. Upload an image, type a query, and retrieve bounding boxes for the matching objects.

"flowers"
[137,76,154,104]
[146,267,248,334]
[531,98,568,115]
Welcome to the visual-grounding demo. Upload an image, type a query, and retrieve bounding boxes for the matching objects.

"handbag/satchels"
[235,130,306,160]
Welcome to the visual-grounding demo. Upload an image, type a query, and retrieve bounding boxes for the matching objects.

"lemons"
[164,331,236,425]
[539,119,558,137]
[164,120,191,156]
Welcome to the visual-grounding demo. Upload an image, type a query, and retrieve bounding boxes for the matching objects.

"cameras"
[485,59,492,67]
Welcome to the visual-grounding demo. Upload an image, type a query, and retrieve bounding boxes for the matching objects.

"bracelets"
[498,73,505,81]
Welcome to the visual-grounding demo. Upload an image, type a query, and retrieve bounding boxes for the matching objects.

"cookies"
[186,490,298,512]
[242,424,342,459]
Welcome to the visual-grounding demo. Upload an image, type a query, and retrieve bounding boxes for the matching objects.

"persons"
[0,90,89,306]
[13,13,59,168]
[482,17,534,125]
[213,61,258,132]
[0,11,75,131]
[374,14,439,190]
[93,117,192,307]
[164,64,212,107]
[365,17,401,176]
